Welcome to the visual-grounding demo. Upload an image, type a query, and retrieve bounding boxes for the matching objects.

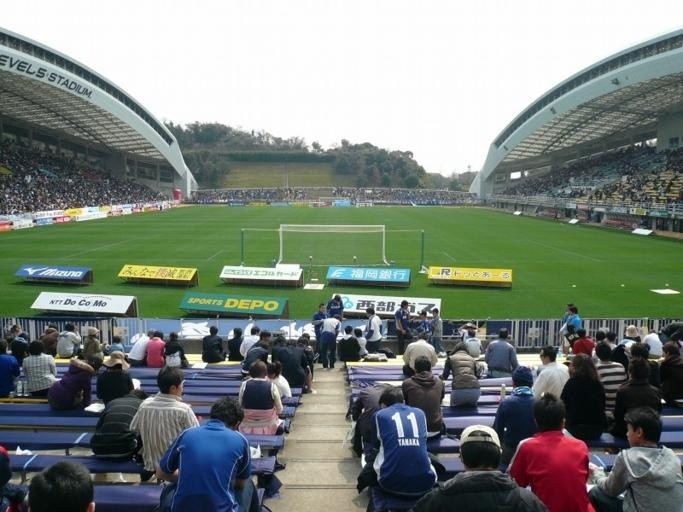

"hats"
[63,324,74,331]
[88,327,100,335]
[460,424,501,448]
[625,325,638,337]
[103,351,130,370]
[512,366,533,384]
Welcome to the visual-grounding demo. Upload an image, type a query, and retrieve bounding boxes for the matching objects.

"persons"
[0,126,171,219]
[0,322,126,413]
[498,139,682,213]
[126,325,315,393]
[90,365,262,512]
[183,186,479,207]
[0,447,96,512]
[349,302,683,511]
[239,360,293,470]
[312,295,442,370]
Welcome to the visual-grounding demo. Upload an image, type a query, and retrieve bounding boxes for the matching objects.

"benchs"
[0,355,302,512]
[345,360,683,512]
